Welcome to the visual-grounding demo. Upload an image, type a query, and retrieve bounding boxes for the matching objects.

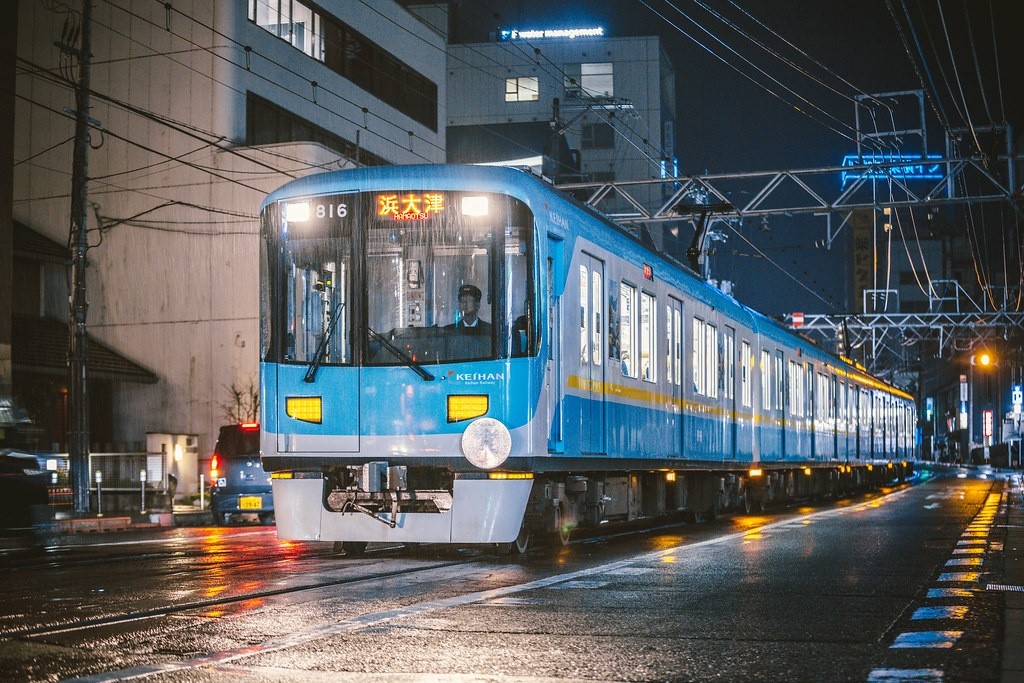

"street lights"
[978,355,1003,446]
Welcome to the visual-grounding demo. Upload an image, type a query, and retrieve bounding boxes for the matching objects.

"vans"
[207,421,275,526]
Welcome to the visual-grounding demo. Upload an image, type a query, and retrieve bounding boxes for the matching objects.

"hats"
[458,284,482,300]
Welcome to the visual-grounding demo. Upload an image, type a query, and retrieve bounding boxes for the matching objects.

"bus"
[254,97,918,560]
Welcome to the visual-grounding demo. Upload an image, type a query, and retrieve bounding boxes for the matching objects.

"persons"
[513,298,528,332]
[206,480,215,509]
[582,340,698,393]
[443,284,493,336]
